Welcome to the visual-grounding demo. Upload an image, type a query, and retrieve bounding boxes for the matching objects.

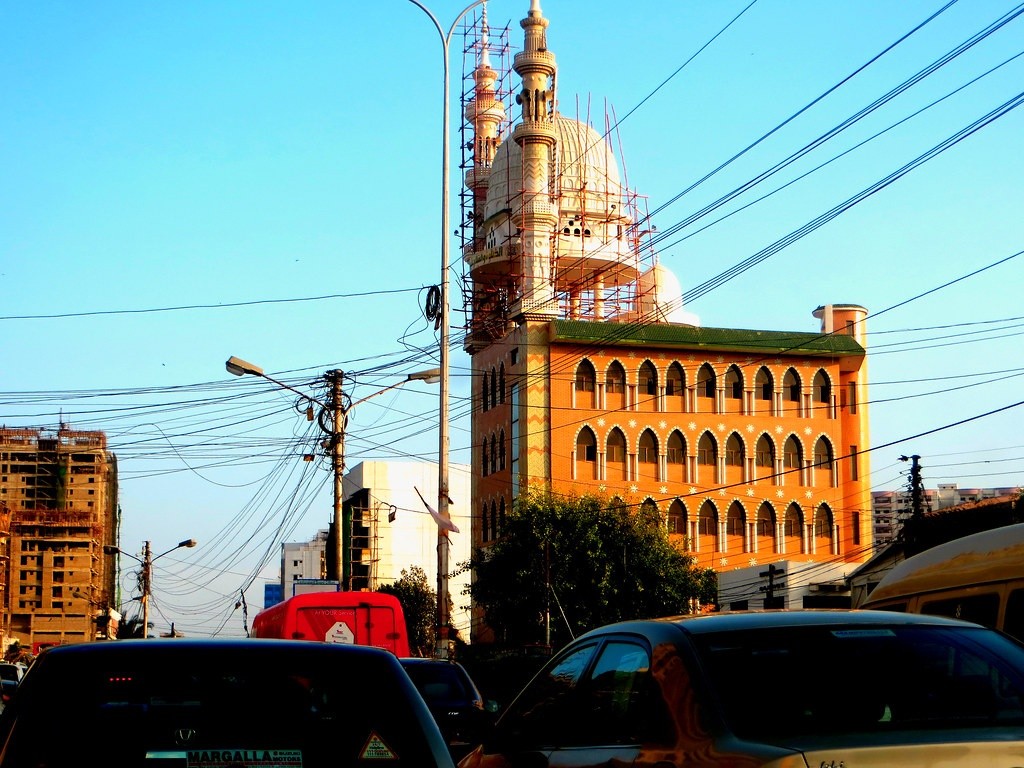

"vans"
[856,522,1023,642]
[246,590,412,658]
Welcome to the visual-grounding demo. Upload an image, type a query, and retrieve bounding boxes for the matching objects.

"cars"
[0,637,456,768]
[455,605,1024,767]
[397,656,485,746]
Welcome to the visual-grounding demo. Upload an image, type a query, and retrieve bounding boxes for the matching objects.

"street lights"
[221,354,440,592]
[73,590,144,640]
[102,538,198,638]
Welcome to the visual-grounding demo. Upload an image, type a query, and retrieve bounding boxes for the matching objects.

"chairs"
[63,704,158,719]
[260,704,348,723]
